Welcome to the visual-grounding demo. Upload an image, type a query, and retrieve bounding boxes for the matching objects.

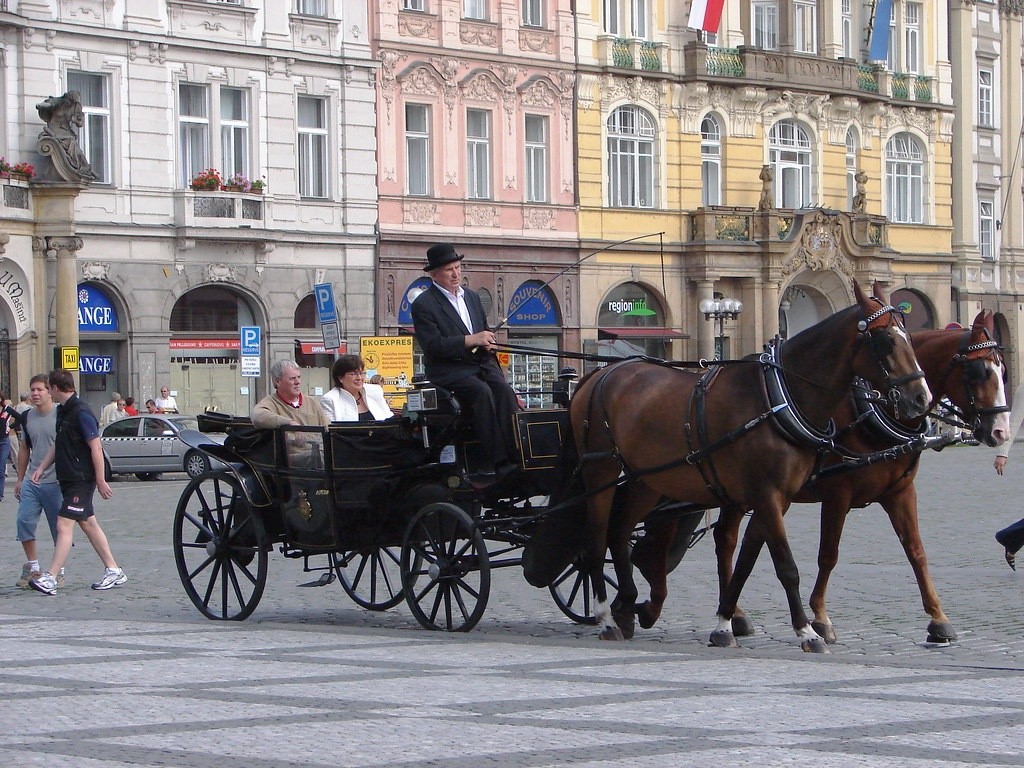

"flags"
[688,0,724,33]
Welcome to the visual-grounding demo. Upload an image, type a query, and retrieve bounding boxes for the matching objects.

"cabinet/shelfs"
[508,353,559,409]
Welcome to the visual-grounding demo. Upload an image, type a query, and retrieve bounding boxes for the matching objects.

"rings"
[995,465,997,468]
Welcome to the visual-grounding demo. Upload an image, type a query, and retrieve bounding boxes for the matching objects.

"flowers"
[192,168,226,191]
[12,162,37,177]
[0,156,12,176]
[226,172,252,192]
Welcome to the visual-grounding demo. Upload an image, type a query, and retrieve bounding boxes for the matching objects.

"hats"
[422,242,465,272]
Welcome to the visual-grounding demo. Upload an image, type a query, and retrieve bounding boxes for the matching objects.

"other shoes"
[995,532,1016,571]
[492,461,519,481]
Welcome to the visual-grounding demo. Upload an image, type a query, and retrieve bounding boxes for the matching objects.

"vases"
[12,174,28,181]
[190,185,216,191]
[0,174,10,179]
[224,185,242,192]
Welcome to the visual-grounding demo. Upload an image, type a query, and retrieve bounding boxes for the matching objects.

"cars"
[98,414,245,481]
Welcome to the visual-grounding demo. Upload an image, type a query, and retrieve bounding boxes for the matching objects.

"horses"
[562,277,1011,655]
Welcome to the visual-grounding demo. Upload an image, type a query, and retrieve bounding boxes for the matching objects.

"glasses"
[347,370,366,378]
[160,390,166,393]
[118,404,126,406]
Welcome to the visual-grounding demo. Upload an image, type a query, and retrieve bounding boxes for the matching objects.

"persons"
[143,399,166,481]
[0,388,37,501]
[14,374,75,589]
[994,383,1024,571]
[320,355,395,424]
[28,369,128,595]
[250,359,329,467]
[758,165,773,210]
[49,90,92,176]
[103,385,178,424]
[411,243,528,482]
[855,170,868,213]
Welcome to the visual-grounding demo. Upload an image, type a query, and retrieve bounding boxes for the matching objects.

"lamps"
[780,285,806,311]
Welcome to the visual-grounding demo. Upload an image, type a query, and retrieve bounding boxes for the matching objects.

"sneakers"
[91,567,128,590]
[54,574,66,588]
[15,564,42,588]
[27,573,58,595]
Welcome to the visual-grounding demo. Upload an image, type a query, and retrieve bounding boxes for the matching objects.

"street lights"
[700,299,744,361]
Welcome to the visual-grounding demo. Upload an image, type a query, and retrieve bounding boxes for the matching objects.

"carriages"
[172,278,1011,654]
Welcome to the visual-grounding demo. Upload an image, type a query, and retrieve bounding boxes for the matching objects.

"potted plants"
[250,179,267,195]
